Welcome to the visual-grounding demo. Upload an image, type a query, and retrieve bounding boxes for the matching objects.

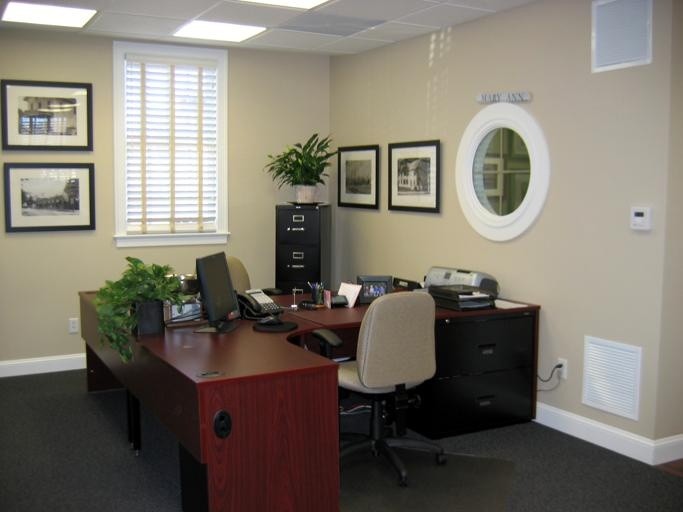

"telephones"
[237,289,283,317]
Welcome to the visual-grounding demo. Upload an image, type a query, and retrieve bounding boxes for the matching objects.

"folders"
[393,278,424,290]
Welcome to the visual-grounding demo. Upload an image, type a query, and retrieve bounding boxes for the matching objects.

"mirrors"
[456,102,552,242]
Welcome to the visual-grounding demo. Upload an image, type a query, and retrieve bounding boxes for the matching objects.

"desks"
[79,290,540,511]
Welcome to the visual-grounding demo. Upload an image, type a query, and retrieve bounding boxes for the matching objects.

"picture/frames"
[4,163,96,232]
[0,79,93,151]
[388,139,441,213]
[337,144,380,209]
[483,128,530,216]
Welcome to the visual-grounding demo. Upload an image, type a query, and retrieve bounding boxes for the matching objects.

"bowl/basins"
[163,274,200,295]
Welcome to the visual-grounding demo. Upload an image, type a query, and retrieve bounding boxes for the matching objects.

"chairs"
[330,291,446,484]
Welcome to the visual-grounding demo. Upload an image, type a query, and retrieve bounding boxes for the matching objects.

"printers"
[413,266,500,300]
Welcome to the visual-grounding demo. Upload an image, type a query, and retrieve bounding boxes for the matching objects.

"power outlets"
[556,359,568,381]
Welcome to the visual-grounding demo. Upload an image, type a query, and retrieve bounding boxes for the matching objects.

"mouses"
[257,316,283,326]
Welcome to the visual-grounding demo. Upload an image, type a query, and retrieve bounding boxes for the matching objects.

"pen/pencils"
[307,281,325,293]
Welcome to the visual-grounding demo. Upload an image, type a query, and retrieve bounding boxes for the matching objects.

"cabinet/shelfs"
[275,205,332,294]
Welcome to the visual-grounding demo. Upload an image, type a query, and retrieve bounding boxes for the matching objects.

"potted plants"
[263,133,337,203]
[93,258,183,364]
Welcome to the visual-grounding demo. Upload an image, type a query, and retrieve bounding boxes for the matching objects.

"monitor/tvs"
[196,251,241,334]
